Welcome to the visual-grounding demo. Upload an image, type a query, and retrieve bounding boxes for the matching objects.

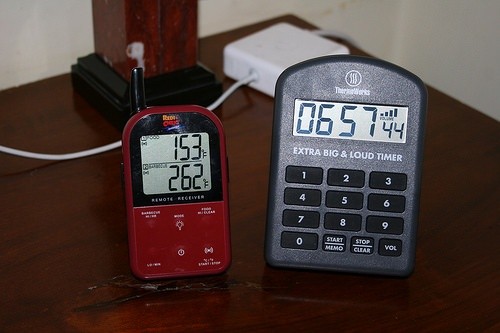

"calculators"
[266,53,427,278]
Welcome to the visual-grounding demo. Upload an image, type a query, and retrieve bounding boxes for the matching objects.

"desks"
[0,13,500,332]
